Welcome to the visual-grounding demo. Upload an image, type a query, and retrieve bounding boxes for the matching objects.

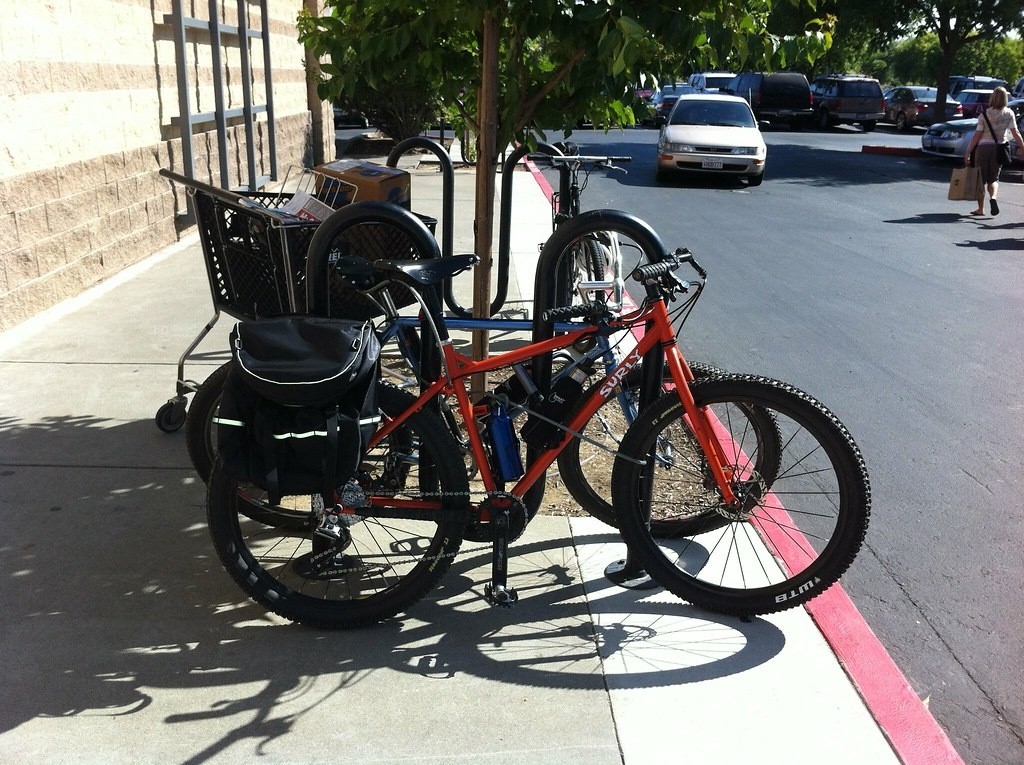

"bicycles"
[527,142,632,351]
[184,254,871,631]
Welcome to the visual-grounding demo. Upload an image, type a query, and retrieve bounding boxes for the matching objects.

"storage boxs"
[314,159,412,212]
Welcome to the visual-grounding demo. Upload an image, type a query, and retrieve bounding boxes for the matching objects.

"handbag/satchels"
[212,317,382,508]
[996,142,1012,165]
[948,163,984,201]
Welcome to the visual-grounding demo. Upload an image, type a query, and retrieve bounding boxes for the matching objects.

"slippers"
[970,211,984,216]
[990,199,999,216]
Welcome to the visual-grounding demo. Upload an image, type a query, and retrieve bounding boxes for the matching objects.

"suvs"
[947,75,1012,100]
[809,74,885,132]
[720,71,814,131]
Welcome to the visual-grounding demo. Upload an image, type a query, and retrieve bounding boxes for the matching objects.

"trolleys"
[157,168,438,432]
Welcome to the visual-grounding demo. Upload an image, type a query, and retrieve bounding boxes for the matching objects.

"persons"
[964,86,1024,216]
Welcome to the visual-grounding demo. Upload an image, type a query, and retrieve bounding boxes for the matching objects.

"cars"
[639,85,700,129]
[688,72,737,94]
[875,86,963,132]
[921,99,1024,166]
[655,94,771,186]
[953,90,1012,118]
[334,100,378,129]
